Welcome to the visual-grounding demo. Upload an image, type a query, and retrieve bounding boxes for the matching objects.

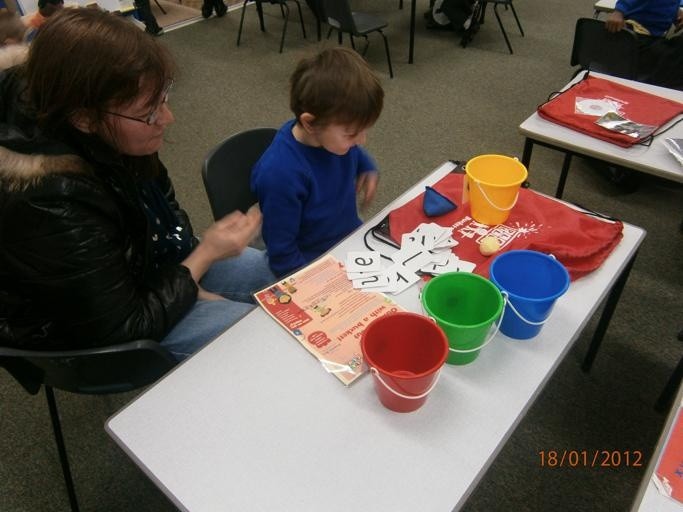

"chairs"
[459,0,525,54]
[571,17,640,83]
[0,340,179,512]
[305,0,394,79]
[236,0,305,53]
[200,127,277,222]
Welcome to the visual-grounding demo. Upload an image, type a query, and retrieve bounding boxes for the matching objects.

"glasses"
[102,77,175,127]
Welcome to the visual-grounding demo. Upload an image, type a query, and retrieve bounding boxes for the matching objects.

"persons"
[202,0,227,18]
[605,0,683,73]
[134,0,163,35]
[440,0,480,48]
[0,5,278,384]
[0,0,64,48]
[249,46,387,279]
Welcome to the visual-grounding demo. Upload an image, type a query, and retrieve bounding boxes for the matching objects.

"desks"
[518,69,683,233]
[593,0,620,20]
[103,160,647,512]
[627,356,683,512]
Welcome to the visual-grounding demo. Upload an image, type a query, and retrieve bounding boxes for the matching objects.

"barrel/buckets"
[489,250,571,340]
[464,155,529,226]
[360,312,449,413]
[421,271,507,366]
